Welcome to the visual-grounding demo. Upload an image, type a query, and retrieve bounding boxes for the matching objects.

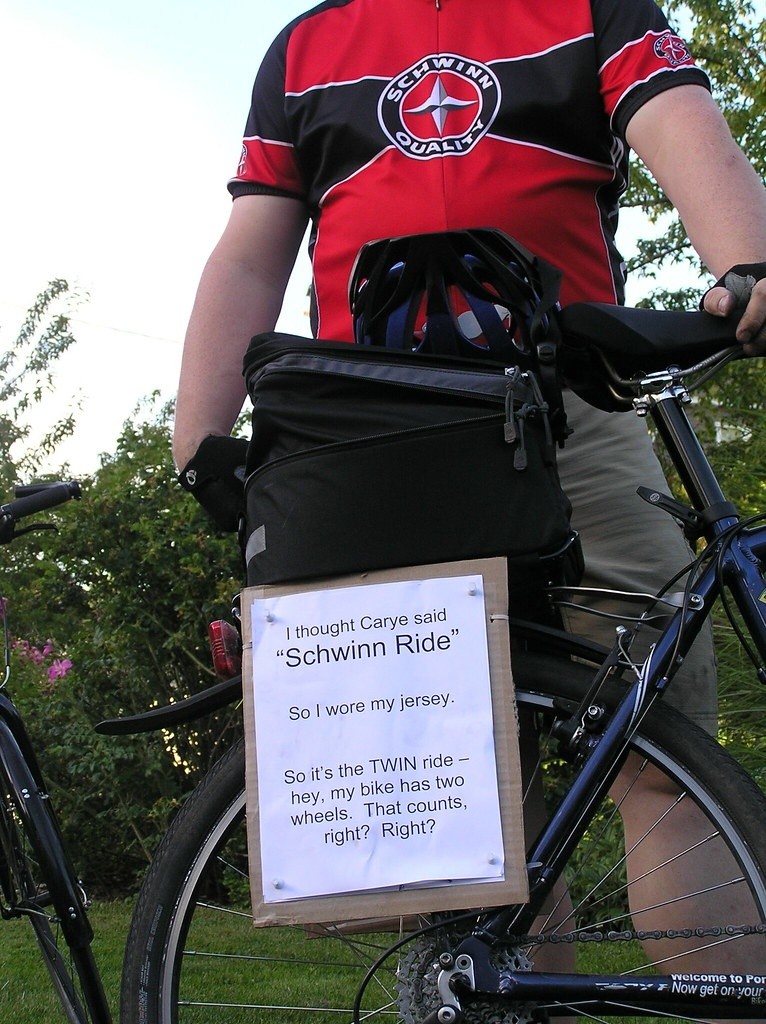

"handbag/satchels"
[178,330,585,616]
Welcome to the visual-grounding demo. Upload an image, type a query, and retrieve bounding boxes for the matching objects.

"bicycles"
[0,477,112,1024]
[94,304,765,1024]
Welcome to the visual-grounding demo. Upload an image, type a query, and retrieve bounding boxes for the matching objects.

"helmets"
[346,227,562,370]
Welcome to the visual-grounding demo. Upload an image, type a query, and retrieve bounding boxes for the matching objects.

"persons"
[171,0,766,979]
